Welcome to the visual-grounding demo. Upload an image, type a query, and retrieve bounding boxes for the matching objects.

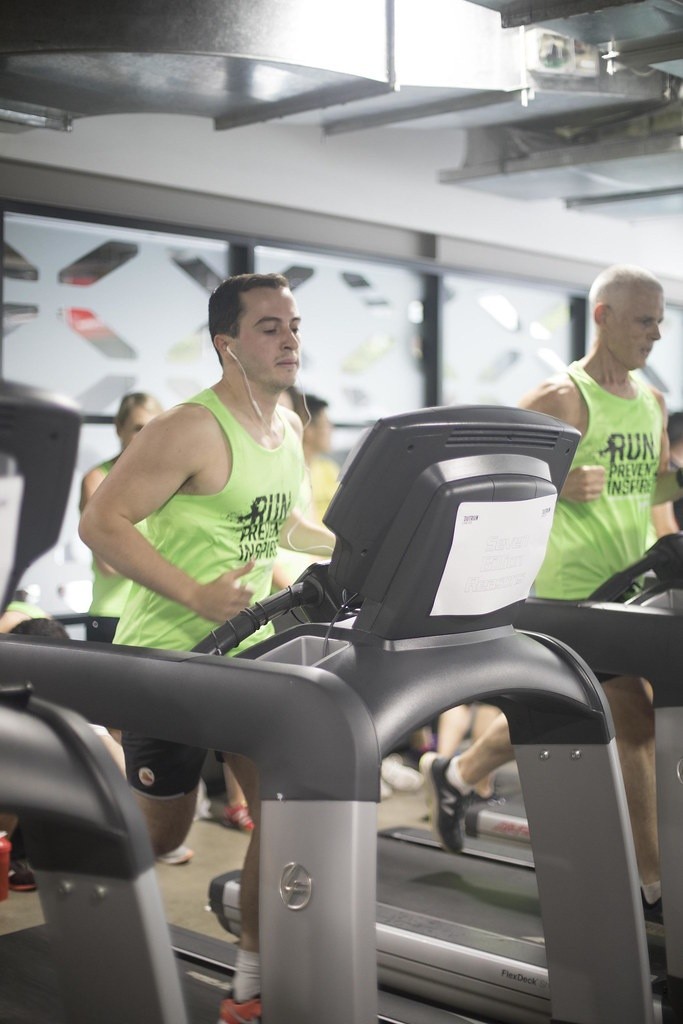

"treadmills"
[0,378,681,1023]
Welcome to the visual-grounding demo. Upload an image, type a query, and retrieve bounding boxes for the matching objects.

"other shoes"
[373,751,424,802]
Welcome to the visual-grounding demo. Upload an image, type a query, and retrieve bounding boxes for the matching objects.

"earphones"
[226,345,238,361]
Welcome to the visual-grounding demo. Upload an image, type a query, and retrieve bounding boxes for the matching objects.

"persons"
[78,394,253,830]
[419,268,683,925]
[271,394,340,597]
[0,273,336,1024]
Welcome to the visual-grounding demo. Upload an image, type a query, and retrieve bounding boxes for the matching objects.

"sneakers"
[418,750,480,853]
[463,787,496,841]
[638,886,666,939]
[218,991,262,1024]
[220,803,254,833]
[156,845,194,866]
[8,858,41,894]
